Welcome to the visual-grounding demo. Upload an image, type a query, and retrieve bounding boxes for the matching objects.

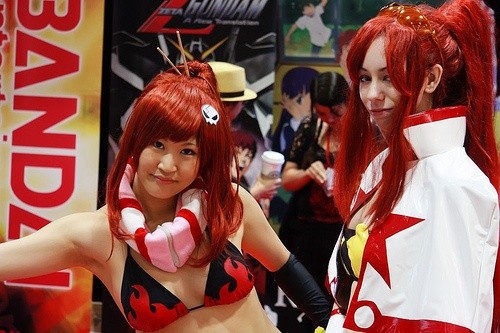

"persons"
[325,1,500,333]
[279,71,351,287]
[206,61,281,204]
[0,61,332,333]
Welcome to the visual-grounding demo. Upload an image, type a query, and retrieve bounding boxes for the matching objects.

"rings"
[272,186,275,190]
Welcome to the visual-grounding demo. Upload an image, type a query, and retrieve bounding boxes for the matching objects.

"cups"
[261,151,285,179]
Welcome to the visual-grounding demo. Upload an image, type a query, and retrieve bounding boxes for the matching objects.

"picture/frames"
[275,0,341,67]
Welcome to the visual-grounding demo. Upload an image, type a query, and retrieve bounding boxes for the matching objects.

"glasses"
[378,2,444,68]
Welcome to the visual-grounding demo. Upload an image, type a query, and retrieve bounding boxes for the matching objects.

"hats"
[207,62,257,101]
